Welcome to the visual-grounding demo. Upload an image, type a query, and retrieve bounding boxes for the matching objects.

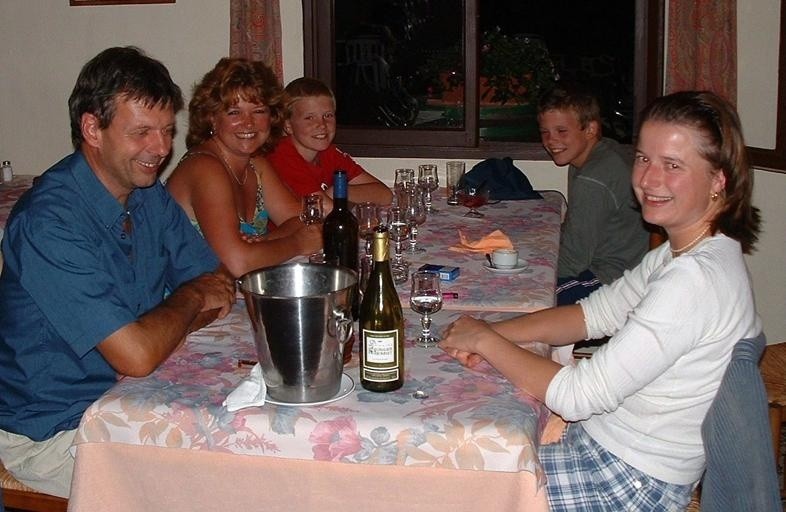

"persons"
[164,57,325,279]
[438,89,766,512]
[264,75,395,233]
[0,44,238,499]
[535,81,651,351]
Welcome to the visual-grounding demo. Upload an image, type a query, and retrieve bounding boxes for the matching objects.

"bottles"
[322,169,357,267]
[357,228,407,396]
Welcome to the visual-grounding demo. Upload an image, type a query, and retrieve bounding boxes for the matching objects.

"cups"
[308,254,323,265]
[492,247,518,268]
[446,161,467,206]
[359,256,374,295]
[299,192,323,224]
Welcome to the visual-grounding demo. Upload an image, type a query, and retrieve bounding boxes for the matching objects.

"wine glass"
[357,163,439,280]
[409,269,443,346]
[453,187,491,218]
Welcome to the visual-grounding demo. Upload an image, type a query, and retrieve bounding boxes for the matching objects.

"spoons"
[486,253,495,269]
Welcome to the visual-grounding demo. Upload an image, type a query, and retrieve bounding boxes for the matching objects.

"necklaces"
[217,142,249,186]
[671,225,711,254]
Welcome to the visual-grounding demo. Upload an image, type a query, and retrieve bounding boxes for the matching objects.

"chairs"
[0,458,68,512]
[685,342,786,512]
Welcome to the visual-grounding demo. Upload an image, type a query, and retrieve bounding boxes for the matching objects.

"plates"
[264,372,355,407]
[482,258,530,275]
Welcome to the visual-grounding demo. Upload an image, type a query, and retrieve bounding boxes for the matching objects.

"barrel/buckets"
[234,262,365,402]
